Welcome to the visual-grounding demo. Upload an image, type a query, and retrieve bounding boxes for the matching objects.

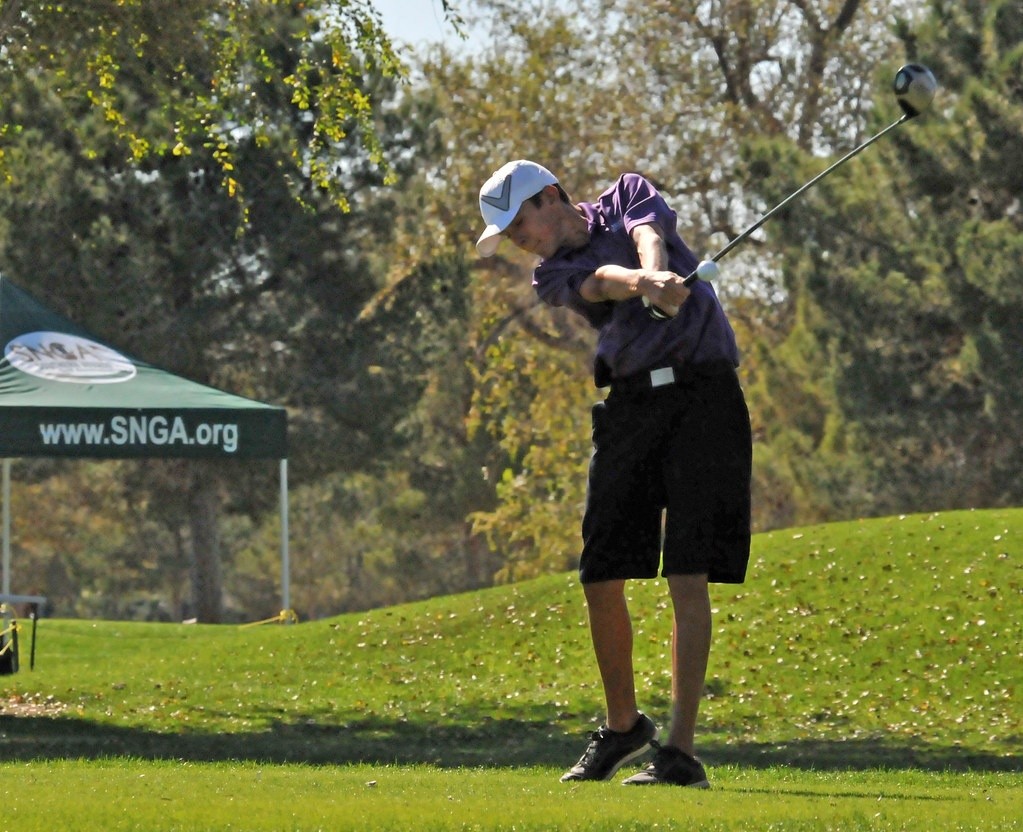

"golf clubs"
[684,62,936,284]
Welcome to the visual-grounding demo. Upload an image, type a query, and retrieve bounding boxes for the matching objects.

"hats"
[475,160,558,258]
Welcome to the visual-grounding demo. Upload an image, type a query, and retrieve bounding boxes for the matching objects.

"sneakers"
[622,740,709,790]
[559,713,658,781]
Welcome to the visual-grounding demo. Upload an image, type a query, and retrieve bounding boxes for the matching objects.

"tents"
[0,274,290,624]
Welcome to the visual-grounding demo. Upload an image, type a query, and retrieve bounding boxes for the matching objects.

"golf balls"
[697,260,718,282]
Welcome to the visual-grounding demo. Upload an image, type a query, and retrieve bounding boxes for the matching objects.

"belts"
[647,364,676,388]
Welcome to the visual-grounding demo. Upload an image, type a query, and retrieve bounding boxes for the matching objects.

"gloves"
[642,294,679,319]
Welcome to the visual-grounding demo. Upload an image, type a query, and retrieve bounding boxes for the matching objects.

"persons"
[474,160,753,786]
